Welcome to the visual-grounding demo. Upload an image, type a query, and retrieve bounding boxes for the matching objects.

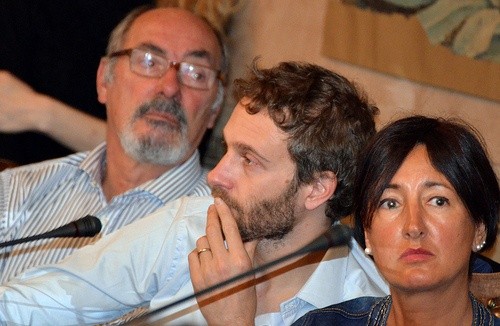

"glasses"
[108,47,224,91]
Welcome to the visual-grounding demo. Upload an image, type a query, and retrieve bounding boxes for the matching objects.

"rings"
[197,248,211,255]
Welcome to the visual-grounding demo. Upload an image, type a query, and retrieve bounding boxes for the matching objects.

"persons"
[0,62,392,326]
[1,1,158,168]
[0,5,230,326]
[288,116,500,326]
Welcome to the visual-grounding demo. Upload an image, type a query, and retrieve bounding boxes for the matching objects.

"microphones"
[119,223,352,326]
[0,214,103,249]
